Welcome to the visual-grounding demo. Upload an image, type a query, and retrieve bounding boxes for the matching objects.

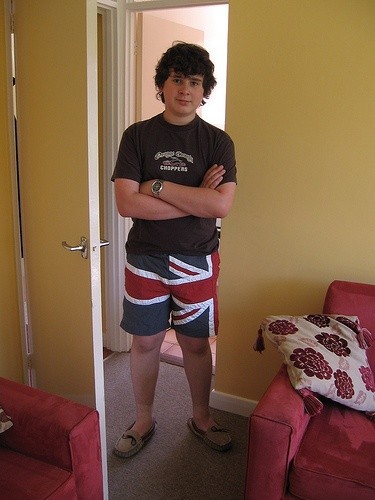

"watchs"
[151,179,166,199]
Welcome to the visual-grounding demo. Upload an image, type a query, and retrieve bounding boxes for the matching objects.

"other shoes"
[114,416,158,457]
[188,417,232,452]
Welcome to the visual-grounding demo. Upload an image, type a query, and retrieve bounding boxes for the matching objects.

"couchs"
[0,376,104,500]
[249,280,375,500]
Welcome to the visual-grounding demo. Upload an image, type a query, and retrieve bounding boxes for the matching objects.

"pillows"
[253,314,375,419]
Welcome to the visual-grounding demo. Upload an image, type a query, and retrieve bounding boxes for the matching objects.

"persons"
[110,44,237,457]
